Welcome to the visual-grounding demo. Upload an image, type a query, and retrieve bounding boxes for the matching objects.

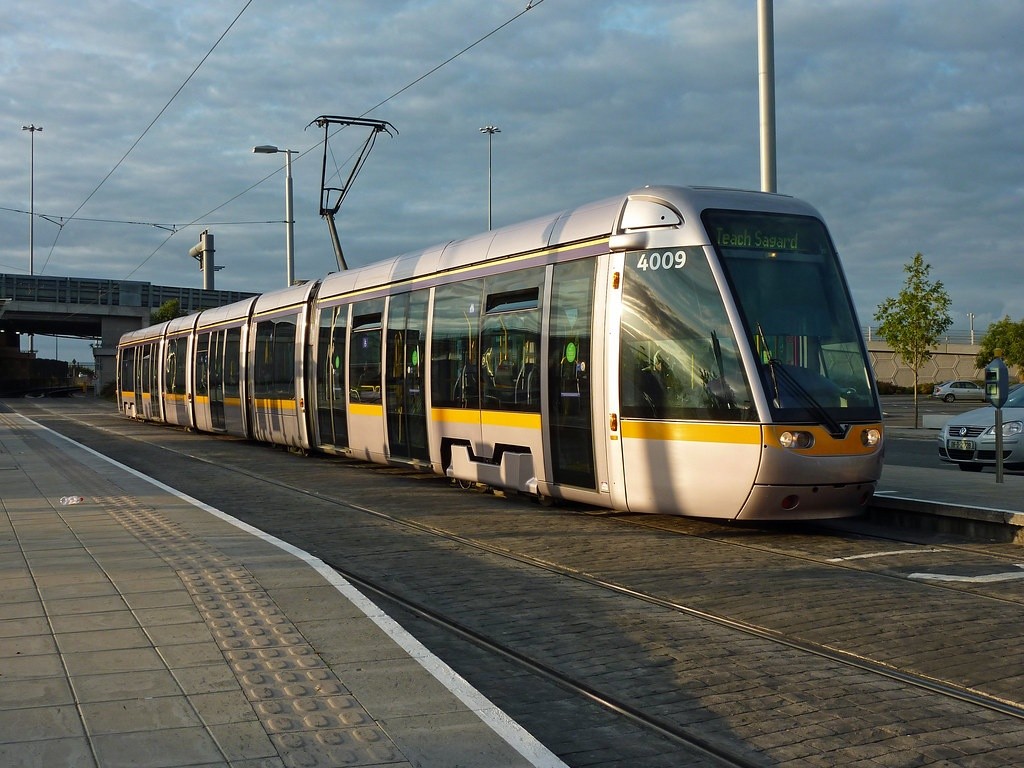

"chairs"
[350,356,581,411]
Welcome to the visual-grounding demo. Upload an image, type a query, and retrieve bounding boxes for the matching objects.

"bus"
[116,185,886,527]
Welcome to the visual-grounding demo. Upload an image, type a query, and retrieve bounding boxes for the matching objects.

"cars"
[933,380,985,403]
[938,384,1024,472]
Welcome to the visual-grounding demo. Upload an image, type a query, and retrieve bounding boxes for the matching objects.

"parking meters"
[985,348,1009,483]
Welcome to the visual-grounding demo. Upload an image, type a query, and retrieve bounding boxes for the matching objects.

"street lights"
[252,145,300,288]
[479,125,501,231]
[21,123,43,275]
